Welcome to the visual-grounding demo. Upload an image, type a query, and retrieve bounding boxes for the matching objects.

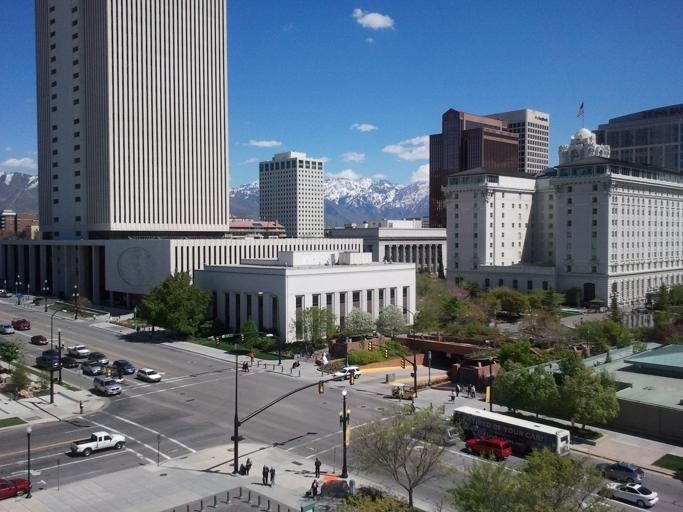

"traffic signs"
[221,333,235,339]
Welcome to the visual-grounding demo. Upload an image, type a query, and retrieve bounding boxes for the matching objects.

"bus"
[450,404,571,460]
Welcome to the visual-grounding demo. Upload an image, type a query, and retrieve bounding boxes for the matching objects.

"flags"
[576,102,584,116]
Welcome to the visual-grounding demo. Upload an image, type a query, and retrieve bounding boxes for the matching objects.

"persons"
[262,465,269,485]
[243,361,246,372]
[246,458,252,475]
[269,466,276,488]
[238,464,246,475]
[450,383,476,402]
[245,361,249,372]
[312,479,319,495]
[315,458,321,478]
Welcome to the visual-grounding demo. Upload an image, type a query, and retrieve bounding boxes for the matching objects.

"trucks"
[390,383,416,400]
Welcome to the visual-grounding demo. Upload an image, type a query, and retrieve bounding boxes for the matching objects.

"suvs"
[332,366,361,381]
[461,437,515,462]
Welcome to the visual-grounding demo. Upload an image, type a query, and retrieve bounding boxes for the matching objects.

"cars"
[27,332,165,398]
[597,479,660,510]
[632,306,649,315]
[0,322,14,335]
[0,289,8,297]
[593,459,645,485]
[10,318,31,331]
[0,477,34,501]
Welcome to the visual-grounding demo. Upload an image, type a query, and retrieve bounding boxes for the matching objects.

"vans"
[420,423,459,447]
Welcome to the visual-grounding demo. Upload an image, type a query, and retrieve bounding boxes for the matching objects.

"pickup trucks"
[69,430,126,458]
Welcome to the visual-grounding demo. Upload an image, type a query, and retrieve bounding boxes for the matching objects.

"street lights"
[230,332,273,476]
[493,309,507,327]
[50,308,67,350]
[345,336,352,366]
[488,356,494,412]
[339,386,351,479]
[388,303,418,397]
[41,278,50,313]
[25,424,33,500]
[255,288,282,365]
[71,283,79,320]
[14,274,22,305]
[54,327,66,386]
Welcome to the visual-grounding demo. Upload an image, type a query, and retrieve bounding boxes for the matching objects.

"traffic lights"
[400,359,405,369]
[349,371,354,385]
[215,336,220,346]
[383,349,387,359]
[368,342,372,351]
[318,380,324,394]
[240,333,245,342]
